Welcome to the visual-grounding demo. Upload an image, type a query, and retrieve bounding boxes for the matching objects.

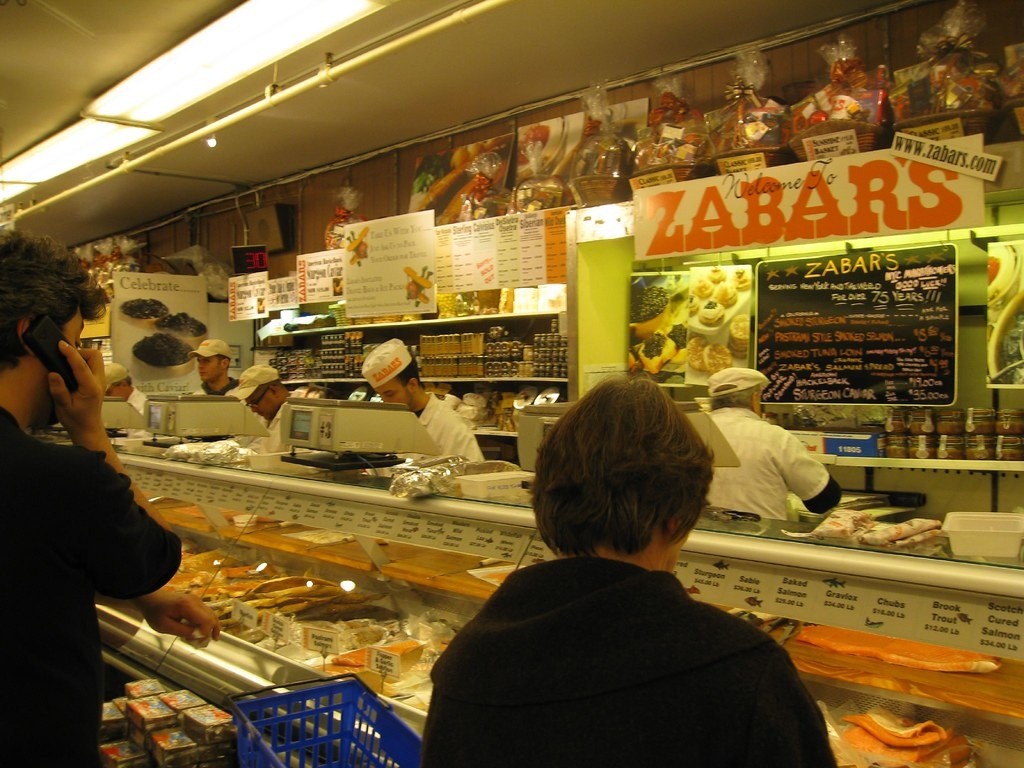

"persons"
[104,338,486,462]
[706,367,842,521]
[420,373,837,768]
[0,228,221,768]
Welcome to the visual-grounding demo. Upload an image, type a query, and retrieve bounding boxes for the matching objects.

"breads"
[638,325,687,374]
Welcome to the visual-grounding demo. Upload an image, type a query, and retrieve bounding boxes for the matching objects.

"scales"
[54,397,147,432]
[281,397,442,457]
[515,398,743,472]
[146,393,273,438]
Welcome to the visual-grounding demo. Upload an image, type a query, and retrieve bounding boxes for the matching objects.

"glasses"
[245,384,277,408]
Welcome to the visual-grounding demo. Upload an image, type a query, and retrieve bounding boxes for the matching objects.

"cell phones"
[22,315,88,394]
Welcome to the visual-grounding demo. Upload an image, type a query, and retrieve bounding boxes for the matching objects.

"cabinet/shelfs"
[281,312,569,436]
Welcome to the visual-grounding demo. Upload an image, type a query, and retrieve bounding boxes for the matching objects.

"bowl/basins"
[129,342,196,382]
[117,310,168,333]
[122,438,144,454]
[245,450,312,472]
[233,515,258,527]
[942,510,1024,558]
[170,330,209,351]
[454,471,536,498]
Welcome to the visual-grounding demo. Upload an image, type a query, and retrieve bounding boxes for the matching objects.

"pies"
[687,314,749,375]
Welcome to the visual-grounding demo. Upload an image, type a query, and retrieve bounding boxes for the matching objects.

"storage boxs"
[941,512,1024,557]
[786,428,823,453]
[453,471,536,497]
[100,679,238,768]
[823,427,885,457]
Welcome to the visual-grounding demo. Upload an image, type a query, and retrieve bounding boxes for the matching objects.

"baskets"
[226,673,424,768]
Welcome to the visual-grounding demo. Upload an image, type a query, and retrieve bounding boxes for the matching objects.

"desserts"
[688,267,750,327]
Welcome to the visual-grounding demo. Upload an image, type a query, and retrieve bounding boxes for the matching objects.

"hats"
[707,368,769,397]
[103,364,128,393]
[229,364,279,400]
[362,338,412,388]
[188,339,232,359]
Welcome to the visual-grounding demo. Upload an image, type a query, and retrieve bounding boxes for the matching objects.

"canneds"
[321,330,367,380]
[418,318,568,379]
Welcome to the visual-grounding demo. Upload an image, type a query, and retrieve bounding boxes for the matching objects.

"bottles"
[319,318,567,378]
[884,408,1024,462]
